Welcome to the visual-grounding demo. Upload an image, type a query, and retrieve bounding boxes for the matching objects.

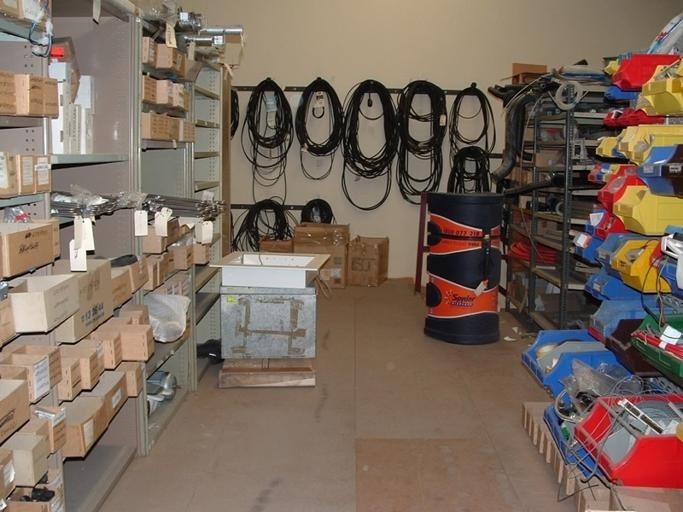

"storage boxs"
[141,238,214,293]
[1,68,60,120]
[63,362,147,459]
[0,150,51,198]
[0,297,19,348]
[0,218,62,279]
[0,0,52,32]
[148,217,183,254]
[261,222,389,286]
[58,300,157,404]
[1,403,70,512]
[61,253,151,345]
[143,32,196,145]
[13,273,79,335]
[1,342,66,446]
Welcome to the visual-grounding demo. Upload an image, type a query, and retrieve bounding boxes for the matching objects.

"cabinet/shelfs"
[0,0,139,512]
[137,17,231,453]
[505,88,607,329]
